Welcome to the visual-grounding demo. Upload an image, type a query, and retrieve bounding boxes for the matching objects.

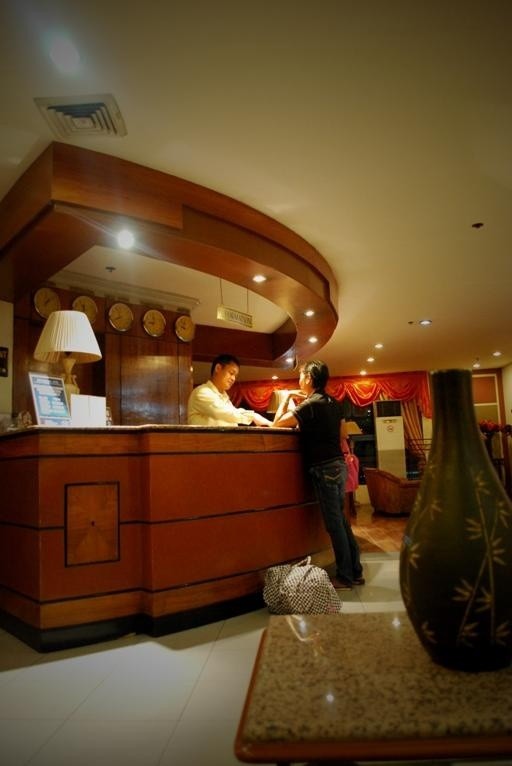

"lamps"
[32,311,102,407]
[345,421,362,454]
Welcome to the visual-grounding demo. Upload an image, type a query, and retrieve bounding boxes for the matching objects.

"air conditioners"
[375,417,406,478]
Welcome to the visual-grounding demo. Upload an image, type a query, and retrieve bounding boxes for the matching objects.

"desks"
[232,613,510,763]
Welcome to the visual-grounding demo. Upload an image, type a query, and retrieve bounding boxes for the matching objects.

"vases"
[485,438,492,459]
[398,370,511,672]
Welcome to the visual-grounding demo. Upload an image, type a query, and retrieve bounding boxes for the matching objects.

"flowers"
[479,418,502,439]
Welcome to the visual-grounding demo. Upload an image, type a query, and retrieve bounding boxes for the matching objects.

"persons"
[272,358,364,590]
[185,351,274,428]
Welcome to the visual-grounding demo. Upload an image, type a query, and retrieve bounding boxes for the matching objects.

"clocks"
[33,287,196,342]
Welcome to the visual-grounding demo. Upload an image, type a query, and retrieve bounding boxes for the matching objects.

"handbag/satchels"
[344,453,359,493]
[263,555,343,615]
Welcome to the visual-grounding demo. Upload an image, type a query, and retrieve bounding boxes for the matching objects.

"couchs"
[363,466,423,516]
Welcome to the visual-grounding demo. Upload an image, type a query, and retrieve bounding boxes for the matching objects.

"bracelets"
[281,399,290,405]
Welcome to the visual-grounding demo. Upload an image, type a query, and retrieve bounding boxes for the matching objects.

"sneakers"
[330,575,351,587]
[349,575,365,585]
